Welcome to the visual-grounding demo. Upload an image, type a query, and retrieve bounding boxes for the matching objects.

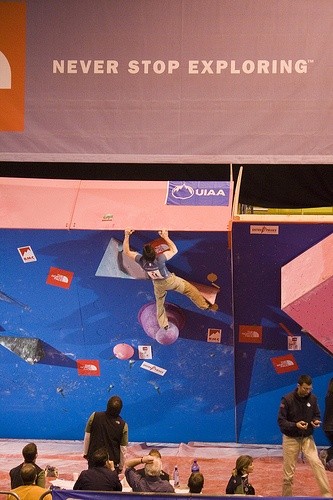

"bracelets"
[141,456,144,463]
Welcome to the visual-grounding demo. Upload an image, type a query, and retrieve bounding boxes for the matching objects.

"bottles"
[174,468,180,486]
[191,461,199,472]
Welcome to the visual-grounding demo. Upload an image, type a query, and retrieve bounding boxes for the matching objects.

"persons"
[276,375,333,497]
[122,226,212,331]
[226,455,256,496]
[9,442,53,500]
[82,396,129,474]
[73,449,204,494]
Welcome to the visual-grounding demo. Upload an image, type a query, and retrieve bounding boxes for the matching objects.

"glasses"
[300,384,312,391]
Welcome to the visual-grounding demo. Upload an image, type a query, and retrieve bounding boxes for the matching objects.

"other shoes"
[211,304,218,312]
[164,325,168,330]
